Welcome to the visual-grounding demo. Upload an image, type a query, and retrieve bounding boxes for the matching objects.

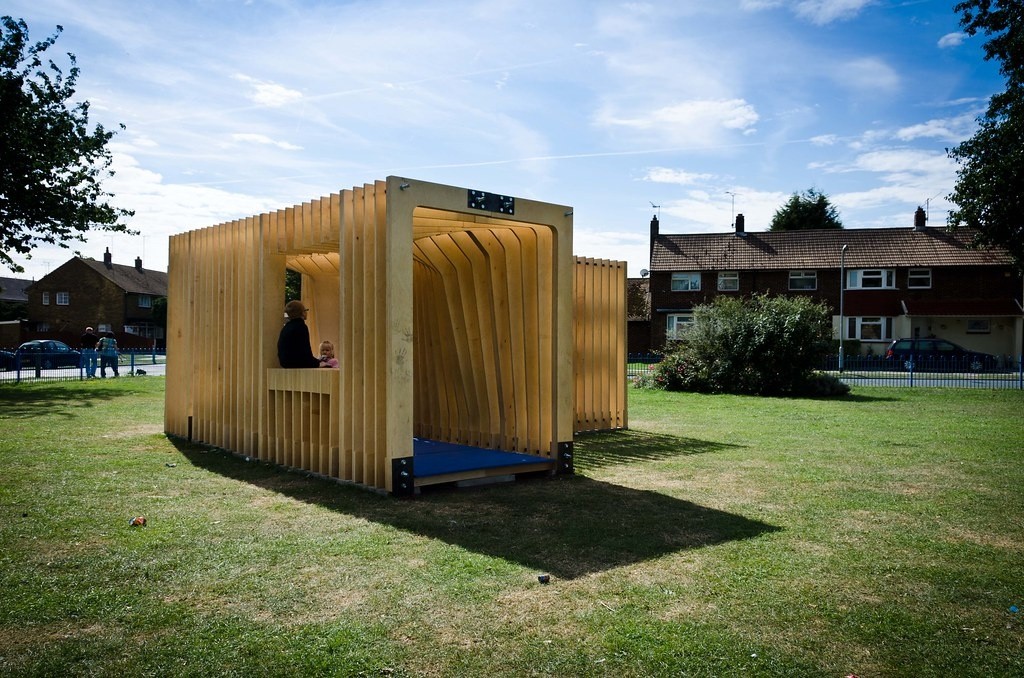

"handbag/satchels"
[97,338,104,352]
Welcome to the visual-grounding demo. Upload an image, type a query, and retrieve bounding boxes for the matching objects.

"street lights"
[838,244,849,374]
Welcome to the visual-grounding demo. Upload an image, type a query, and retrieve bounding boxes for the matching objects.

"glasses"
[302,309,309,312]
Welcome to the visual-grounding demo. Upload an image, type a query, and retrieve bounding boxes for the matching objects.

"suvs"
[884,337,997,374]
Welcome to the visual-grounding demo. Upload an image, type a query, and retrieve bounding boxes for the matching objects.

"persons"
[319,341,338,367]
[80,327,99,379]
[277,301,331,367]
[95,331,119,378]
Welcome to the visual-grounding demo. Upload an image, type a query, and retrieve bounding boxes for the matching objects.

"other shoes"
[91,374,95,377]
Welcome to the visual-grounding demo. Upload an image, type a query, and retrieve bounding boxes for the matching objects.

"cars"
[0,350,16,371]
[15,338,85,370]
